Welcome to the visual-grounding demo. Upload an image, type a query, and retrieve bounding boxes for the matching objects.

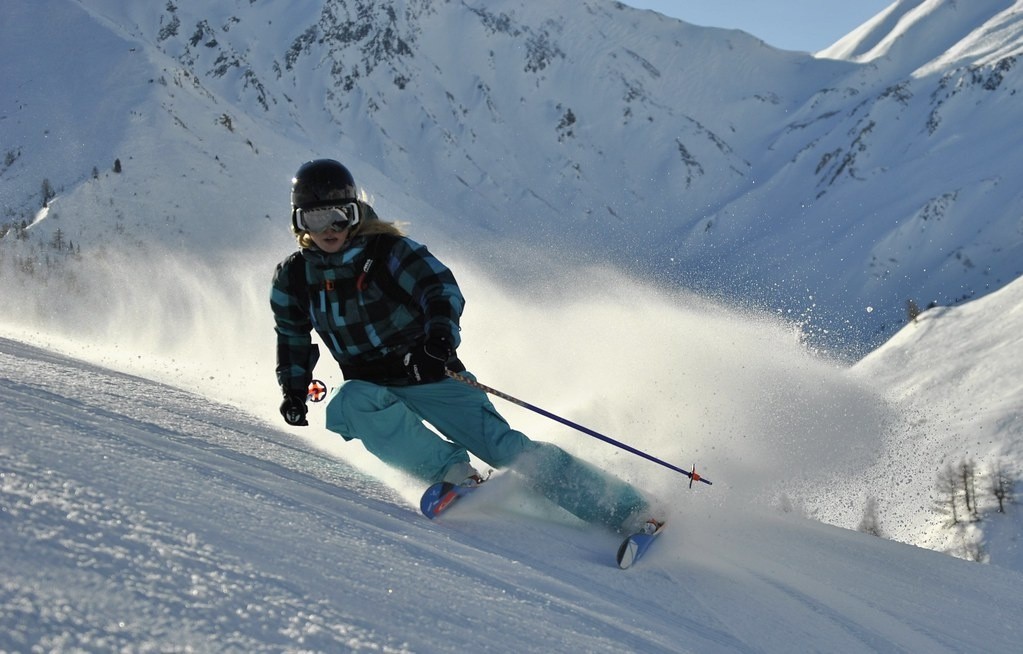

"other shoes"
[463,475,483,488]
[641,517,666,537]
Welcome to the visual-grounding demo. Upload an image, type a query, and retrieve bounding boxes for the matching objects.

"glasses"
[291,203,361,234]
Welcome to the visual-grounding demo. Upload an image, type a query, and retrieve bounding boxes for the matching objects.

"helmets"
[290,158,358,208]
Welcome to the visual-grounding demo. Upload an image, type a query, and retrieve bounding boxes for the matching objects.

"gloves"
[279,394,309,426]
[416,330,454,374]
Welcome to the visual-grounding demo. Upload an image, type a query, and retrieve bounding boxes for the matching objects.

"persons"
[269,159,650,535]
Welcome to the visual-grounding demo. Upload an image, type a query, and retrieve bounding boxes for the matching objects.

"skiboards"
[419,467,674,570]
